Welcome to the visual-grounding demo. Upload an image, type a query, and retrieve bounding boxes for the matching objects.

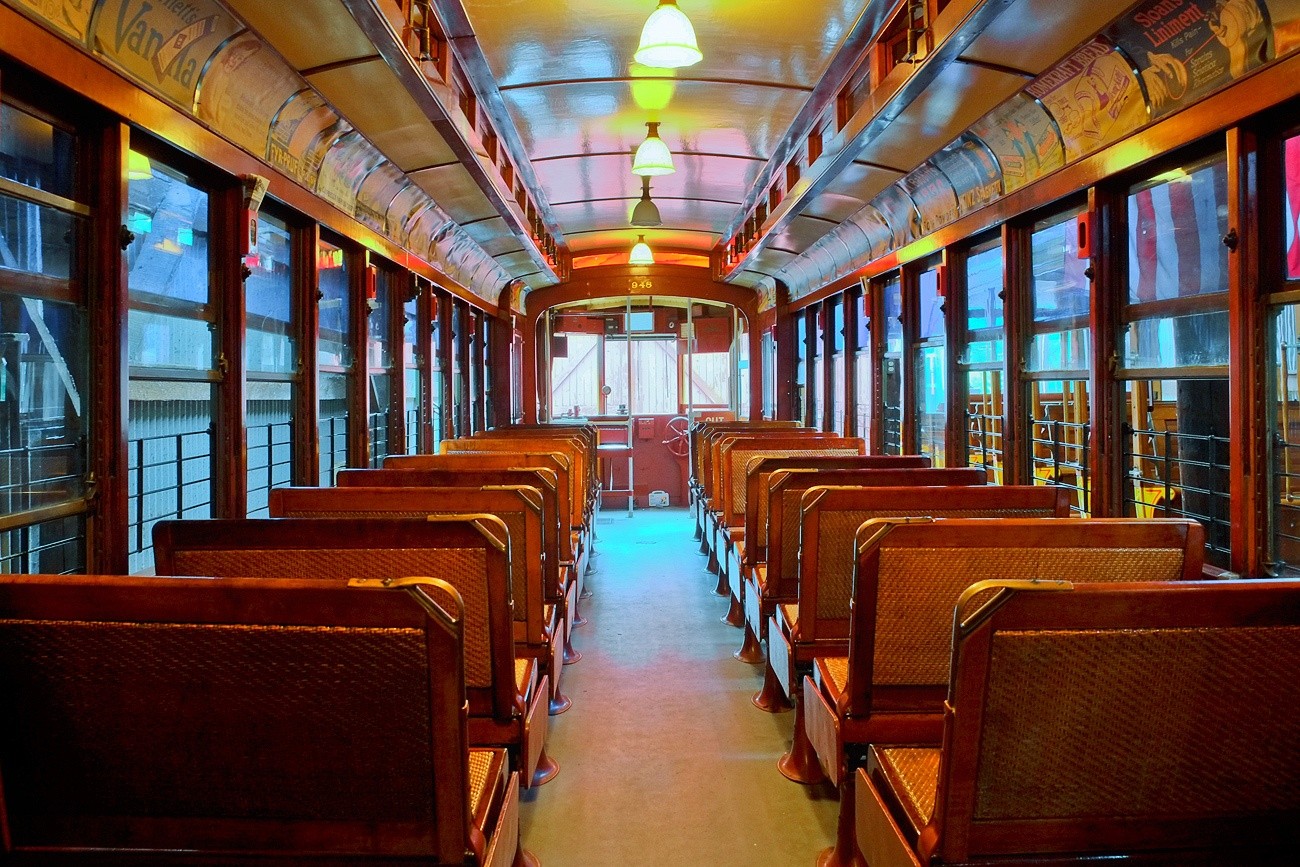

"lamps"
[633,0,703,69]
[629,187,664,226]
[631,121,677,176]
[627,235,655,264]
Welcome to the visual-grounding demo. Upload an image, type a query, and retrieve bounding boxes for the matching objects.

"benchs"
[686,342,1300,867]
[0,418,602,867]
